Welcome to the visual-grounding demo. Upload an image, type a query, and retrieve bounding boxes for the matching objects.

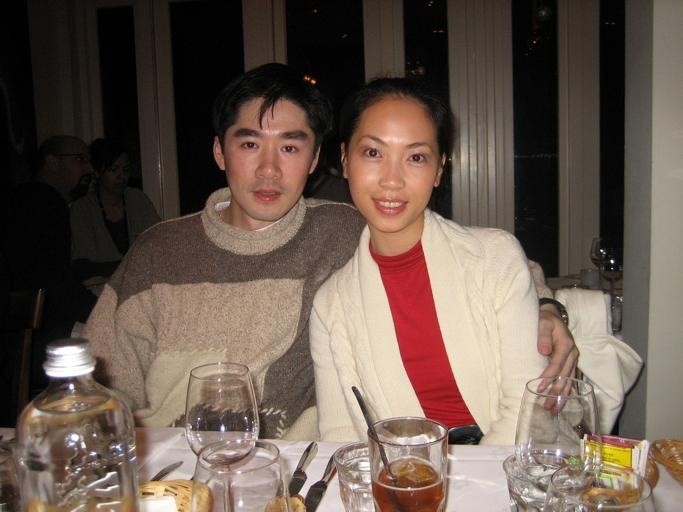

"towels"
[551,286,643,439]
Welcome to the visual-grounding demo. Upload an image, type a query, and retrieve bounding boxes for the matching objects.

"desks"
[543,273,623,336]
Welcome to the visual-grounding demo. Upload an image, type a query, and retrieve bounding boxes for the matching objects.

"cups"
[192,439,293,512]
[601,254,622,297]
[545,461,655,508]
[590,237,611,271]
[367,418,449,507]
[504,453,572,512]
[185,361,260,512]
[336,442,380,508]
[515,377,601,462]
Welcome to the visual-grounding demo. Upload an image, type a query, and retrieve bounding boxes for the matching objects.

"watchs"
[539,298,570,327]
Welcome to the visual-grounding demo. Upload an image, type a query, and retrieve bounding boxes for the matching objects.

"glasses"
[53,153,92,165]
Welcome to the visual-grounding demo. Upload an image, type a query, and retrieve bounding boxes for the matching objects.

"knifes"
[289,440,318,495]
[304,447,337,512]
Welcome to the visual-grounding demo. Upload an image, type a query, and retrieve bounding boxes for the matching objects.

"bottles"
[16,337,140,512]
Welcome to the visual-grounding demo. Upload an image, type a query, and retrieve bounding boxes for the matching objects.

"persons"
[83,63,576,442]
[70,137,158,262]
[0,134,97,323]
[309,77,552,445]
